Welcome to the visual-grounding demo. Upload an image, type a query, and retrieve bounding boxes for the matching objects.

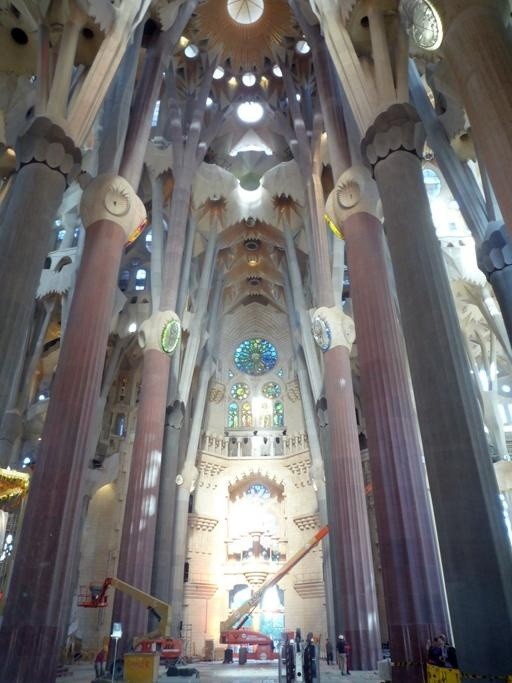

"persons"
[95,648,106,677]
[325,635,351,675]
[437,634,459,670]
[428,638,444,667]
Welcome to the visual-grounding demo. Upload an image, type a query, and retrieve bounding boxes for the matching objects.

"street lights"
[109,620,122,682]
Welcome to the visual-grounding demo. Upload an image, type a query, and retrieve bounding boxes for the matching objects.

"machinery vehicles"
[78,578,182,668]
[218,482,375,664]
